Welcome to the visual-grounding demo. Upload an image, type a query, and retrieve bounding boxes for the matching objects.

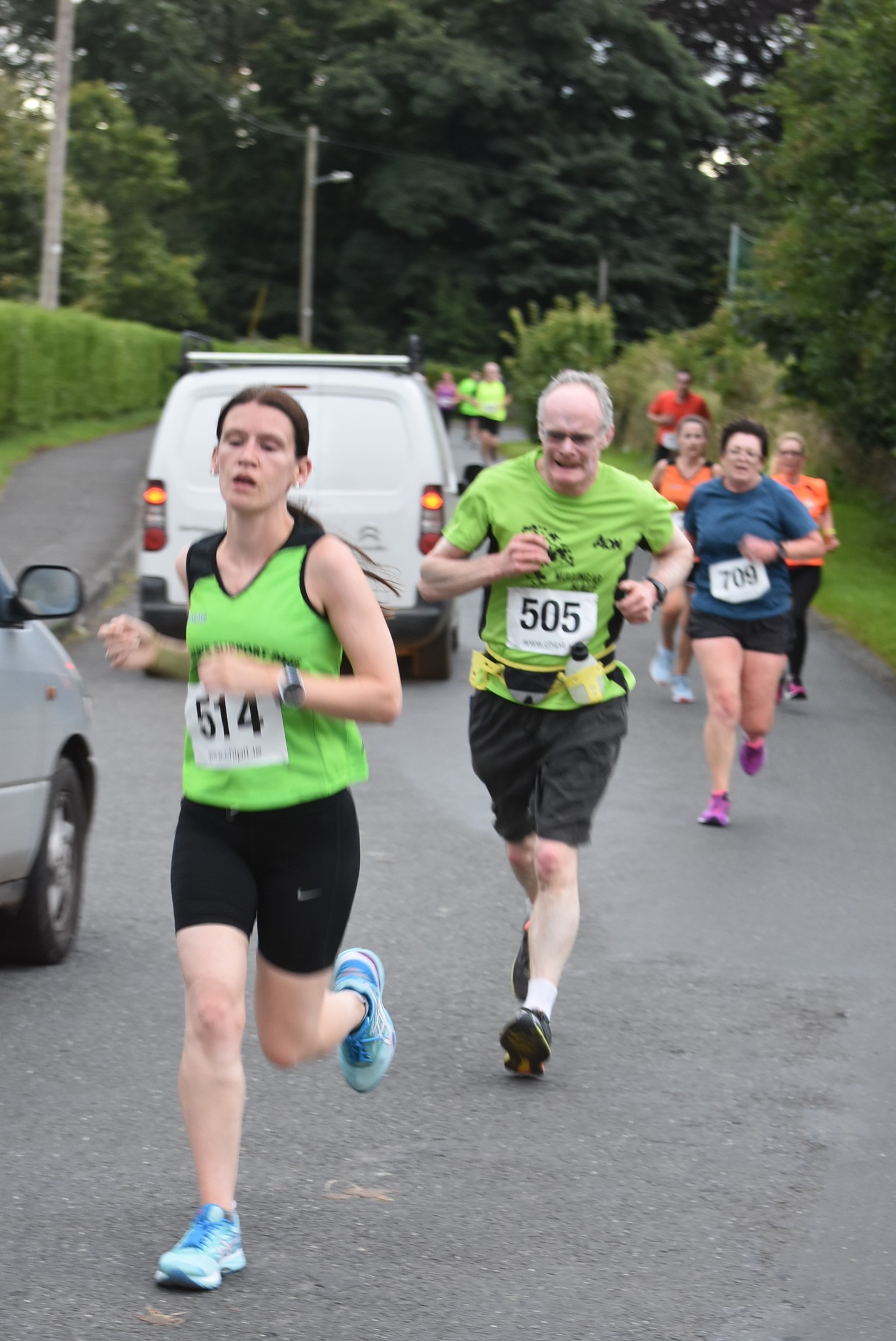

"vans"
[137,350,483,684]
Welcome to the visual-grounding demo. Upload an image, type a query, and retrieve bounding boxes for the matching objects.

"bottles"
[565,643,606,703]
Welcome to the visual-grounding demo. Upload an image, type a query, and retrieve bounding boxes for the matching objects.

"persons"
[97,388,401,1290]
[650,418,723,705]
[419,371,692,1077]
[771,433,838,703]
[683,420,825,826]
[647,369,711,464]
[436,362,511,464]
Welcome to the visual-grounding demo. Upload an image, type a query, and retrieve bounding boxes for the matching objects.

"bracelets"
[823,527,837,535]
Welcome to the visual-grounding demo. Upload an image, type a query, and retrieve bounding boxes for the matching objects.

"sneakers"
[670,676,694,703]
[738,731,764,777]
[152,1204,246,1289]
[775,673,785,704]
[331,947,397,1095]
[649,645,671,686]
[697,789,732,827]
[497,1006,554,1078]
[508,911,535,1002]
[781,674,807,700]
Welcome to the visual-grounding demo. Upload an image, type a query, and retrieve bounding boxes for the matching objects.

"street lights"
[298,170,356,354]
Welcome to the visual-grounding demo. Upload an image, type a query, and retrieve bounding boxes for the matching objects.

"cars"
[0,555,98,970]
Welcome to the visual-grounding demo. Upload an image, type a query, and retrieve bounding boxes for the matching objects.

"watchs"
[276,665,304,705]
[644,575,667,609]
[774,540,786,561]
